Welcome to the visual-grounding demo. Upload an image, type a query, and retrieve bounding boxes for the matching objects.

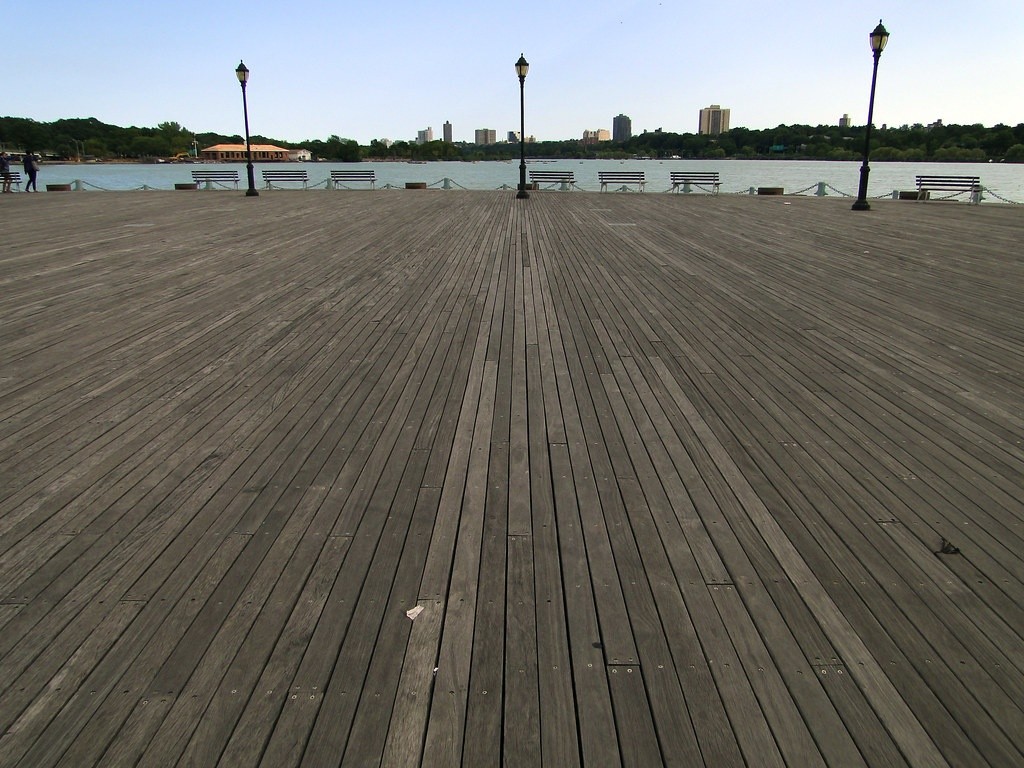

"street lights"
[850,19,889,211]
[512,52,532,201]
[234,59,258,197]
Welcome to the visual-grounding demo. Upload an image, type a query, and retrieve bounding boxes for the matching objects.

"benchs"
[669,171,723,197]
[598,171,648,195]
[916,175,983,205]
[529,171,578,193]
[191,171,241,191]
[330,170,378,192]
[262,170,310,191]
[0,172,23,193]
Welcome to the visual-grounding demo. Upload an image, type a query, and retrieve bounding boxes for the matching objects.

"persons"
[23,150,40,192]
[0,152,12,192]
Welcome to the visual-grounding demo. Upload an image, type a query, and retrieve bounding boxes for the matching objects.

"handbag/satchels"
[31,155,40,171]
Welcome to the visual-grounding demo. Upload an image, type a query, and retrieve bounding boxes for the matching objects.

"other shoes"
[25,189,30,192]
[34,191,38,192]
